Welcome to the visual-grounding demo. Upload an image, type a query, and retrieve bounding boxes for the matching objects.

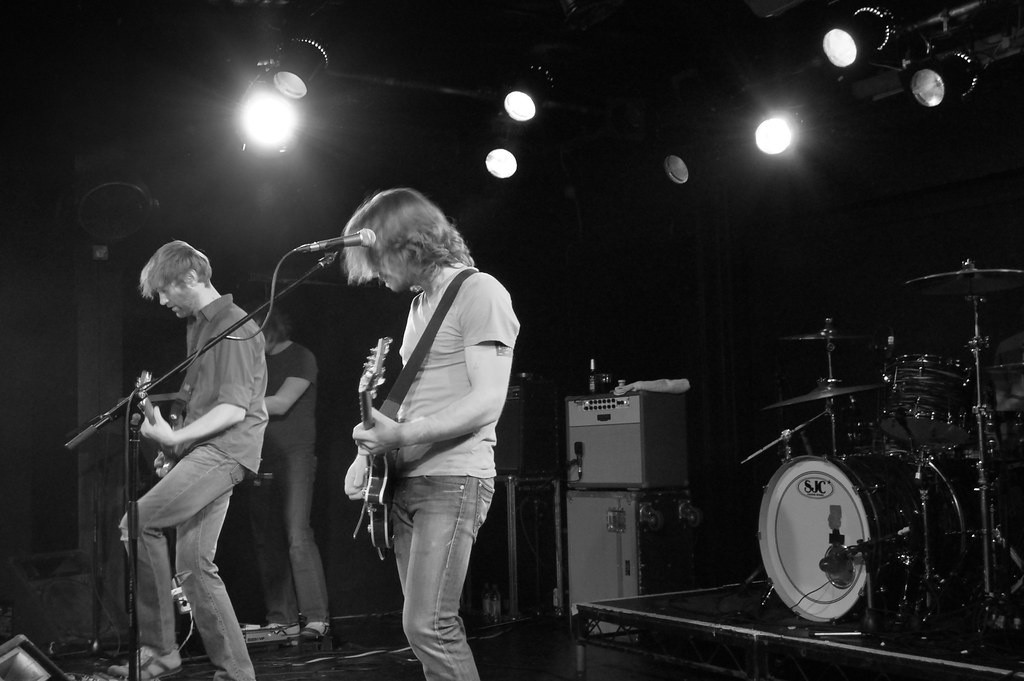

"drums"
[878,352,975,450]
[935,451,1000,538]
[757,447,966,624]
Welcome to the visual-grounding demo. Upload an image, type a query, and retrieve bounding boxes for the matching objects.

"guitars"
[364,335,394,550]
[135,369,185,478]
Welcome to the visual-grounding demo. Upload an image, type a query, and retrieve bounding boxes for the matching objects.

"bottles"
[587,358,597,394]
[481,583,502,624]
[615,380,626,389]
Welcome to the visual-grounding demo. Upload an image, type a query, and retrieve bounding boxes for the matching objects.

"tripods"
[49,418,125,668]
[887,287,1024,654]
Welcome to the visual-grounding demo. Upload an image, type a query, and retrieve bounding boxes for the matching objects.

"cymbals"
[761,382,885,412]
[903,267,1024,295]
[777,330,873,341]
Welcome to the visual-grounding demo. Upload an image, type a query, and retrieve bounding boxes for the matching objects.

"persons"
[341,188,522,681]
[244,302,330,640]
[108,240,269,680]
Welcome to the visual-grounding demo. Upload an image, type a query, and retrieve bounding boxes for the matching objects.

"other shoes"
[300,621,329,637]
[262,622,301,637]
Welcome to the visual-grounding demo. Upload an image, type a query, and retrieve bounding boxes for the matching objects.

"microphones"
[295,228,376,253]
[574,440,583,480]
[819,556,845,574]
[886,329,895,360]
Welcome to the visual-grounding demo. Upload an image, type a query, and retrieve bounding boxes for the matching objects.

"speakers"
[493,382,567,479]
[565,391,690,492]
[571,488,690,636]
[0,634,75,681]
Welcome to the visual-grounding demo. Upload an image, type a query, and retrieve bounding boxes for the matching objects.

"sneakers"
[108,645,184,681]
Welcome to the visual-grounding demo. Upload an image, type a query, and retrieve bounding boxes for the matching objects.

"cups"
[596,373,612,394]
